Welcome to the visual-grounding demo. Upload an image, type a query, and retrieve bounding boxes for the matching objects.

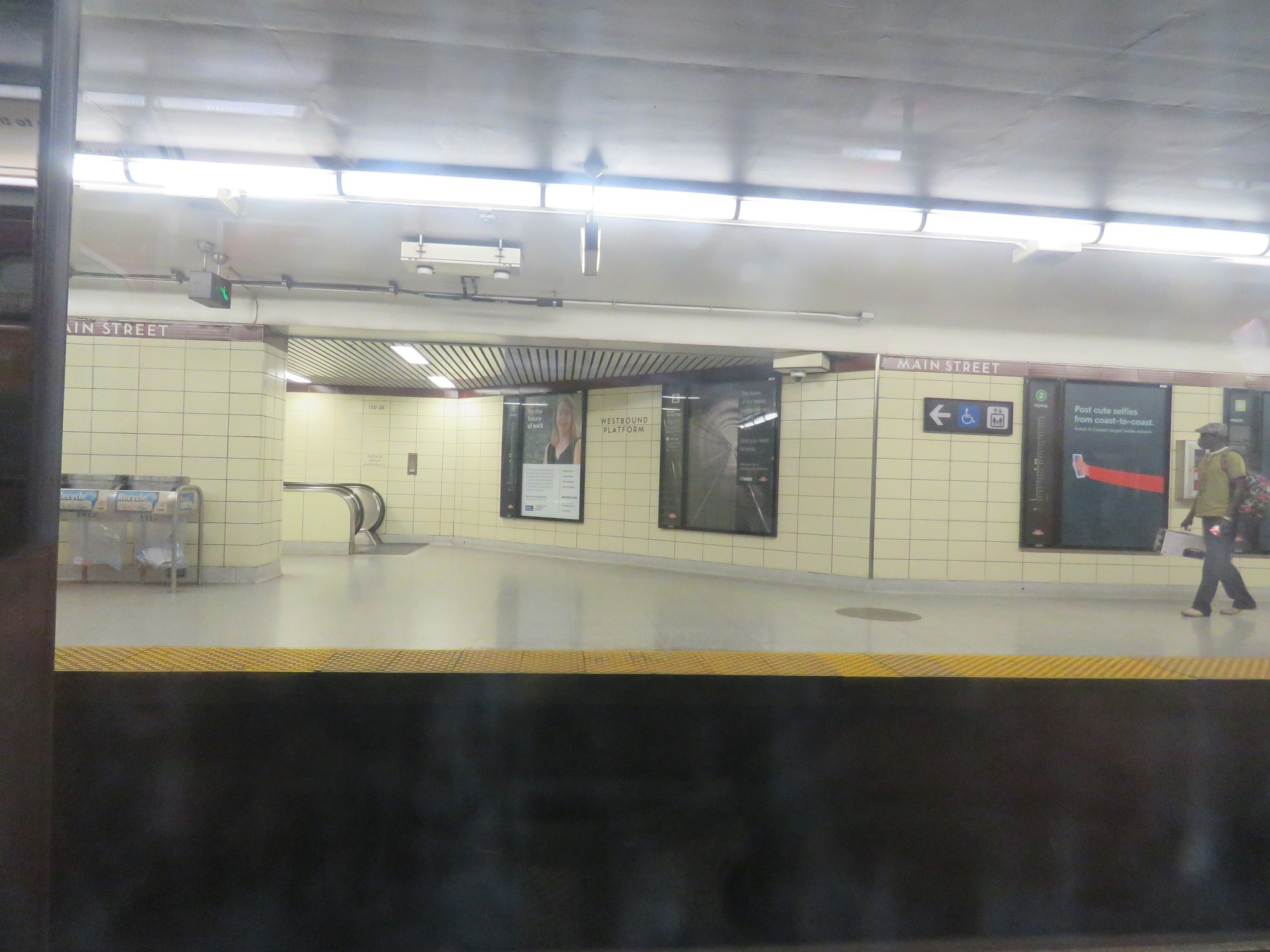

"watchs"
[1223,515,1233,522]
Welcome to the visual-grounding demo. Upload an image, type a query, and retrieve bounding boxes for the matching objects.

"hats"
[1194,422,1228,436]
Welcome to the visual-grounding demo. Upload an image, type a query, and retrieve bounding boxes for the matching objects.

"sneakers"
[1181,607,1203,617]
[1220,606,1244,615]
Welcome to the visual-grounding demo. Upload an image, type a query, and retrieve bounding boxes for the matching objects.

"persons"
[542,395,582,464]
[1179,421,1258,618]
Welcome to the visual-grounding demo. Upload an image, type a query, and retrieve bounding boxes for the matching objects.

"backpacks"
[1220,448,1270,525]
